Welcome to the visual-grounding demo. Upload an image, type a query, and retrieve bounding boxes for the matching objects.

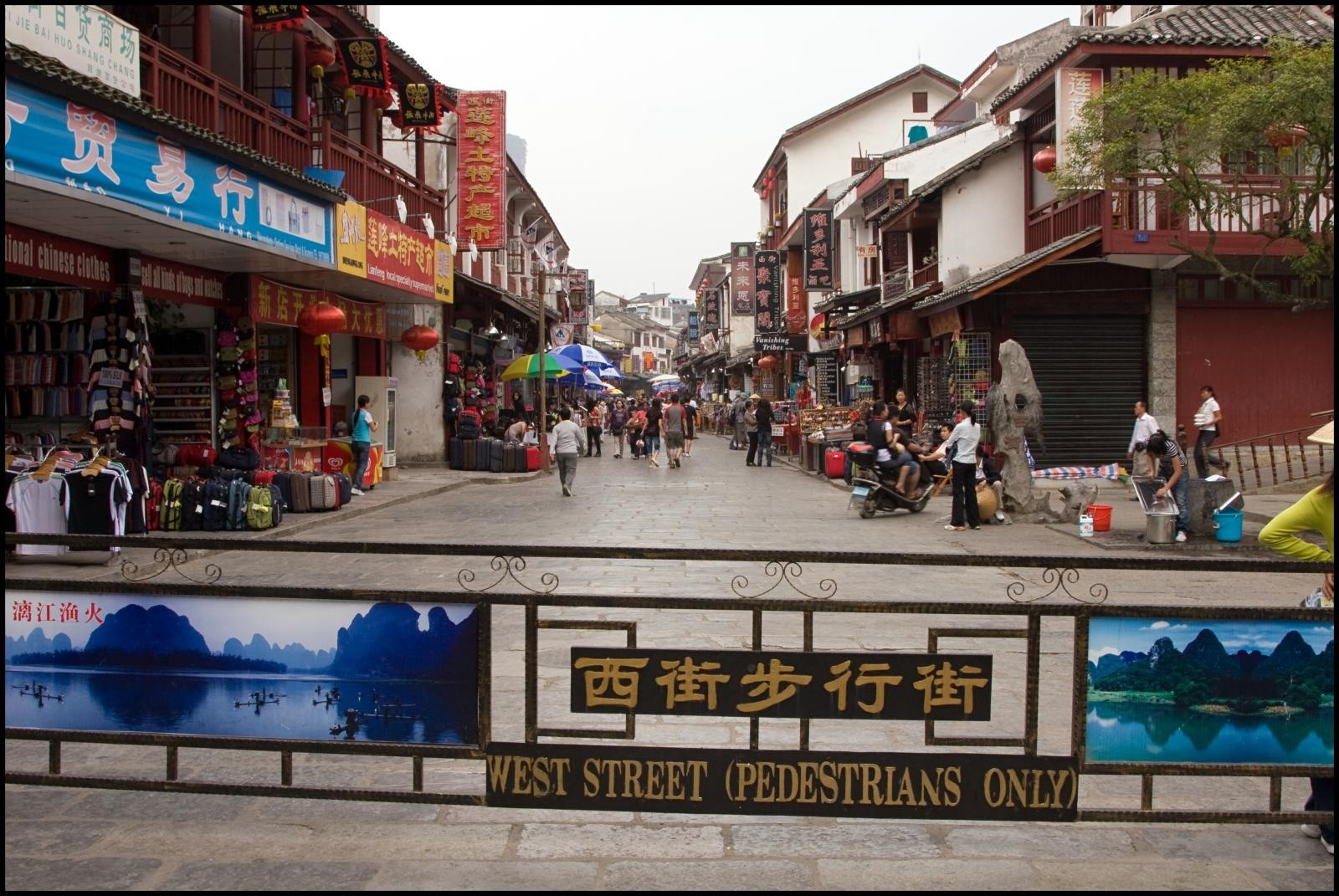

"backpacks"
[146,476,284,532]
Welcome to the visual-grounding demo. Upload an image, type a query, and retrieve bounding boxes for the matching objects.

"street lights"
[537,270,587,472]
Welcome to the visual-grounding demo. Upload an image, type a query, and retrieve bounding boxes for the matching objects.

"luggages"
[448,415,542,472]
[199,463,352,512]
[825,450,845,479]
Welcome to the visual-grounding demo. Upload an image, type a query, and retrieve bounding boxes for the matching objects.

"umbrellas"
[652,374,683,390]
[499,343,626,393]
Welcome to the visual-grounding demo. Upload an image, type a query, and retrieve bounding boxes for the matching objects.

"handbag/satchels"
[612,425,624,436]
[445,352,496,435]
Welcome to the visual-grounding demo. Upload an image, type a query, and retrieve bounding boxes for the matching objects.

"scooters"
[847,440,937,519]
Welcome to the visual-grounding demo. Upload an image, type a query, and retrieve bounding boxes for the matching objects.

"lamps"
[440,239,478,262]
[413,228,457,256]
[482,302,499,335]
[500,322,509,340]
[389,212,435,239]
[358,194,407,224]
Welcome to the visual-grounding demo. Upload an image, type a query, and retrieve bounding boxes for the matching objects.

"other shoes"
[1176,531,1187,542]
[907,488,923,499]
[648,464,653,468]
[729,445,772,467]
[685,452,692,457]
[681,451,686,456]
[944,523,965,530]
[896,485,906,494]
[350,487,365,496]
[671,462,675,468]
[563,484,571,497]
[593,453,601,457]
[614,454,618,458]
[674,457,680,467]
[962,521,981,529]
[633,456,640,460]
[651,458,659,467]
[584,453,592,457]
[1222,461,1231,477]
[995,509,1005,521]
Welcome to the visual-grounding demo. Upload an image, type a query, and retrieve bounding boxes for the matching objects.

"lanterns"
[1033,146,1056,174]
[763,169,775,198]
[752,376,758,383]
[758,354,777,371]
[298,302,346,356]
[401,324,440,361]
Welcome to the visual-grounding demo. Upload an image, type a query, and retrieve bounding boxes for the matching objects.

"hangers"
[5,446,16,469]
[30,446,60,482]
[81,449,111,476]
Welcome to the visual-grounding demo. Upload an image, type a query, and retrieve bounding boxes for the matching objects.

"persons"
[549,409,585,497]
[1194,385,1230,479]
[567,394,700,469]
[729,393,774,467]
[1258,422,1334,855]
[944,400,1004,530]
[351,394,378,495]
[1128,400,1159,501]
[1147,429,1189,542]
[864,390,952,500]
[504,392,529,444]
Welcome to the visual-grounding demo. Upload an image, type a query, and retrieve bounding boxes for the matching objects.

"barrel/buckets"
[1212,508,1245,542]
[1086,505,1113,531]
[1146,514,1176,544]
